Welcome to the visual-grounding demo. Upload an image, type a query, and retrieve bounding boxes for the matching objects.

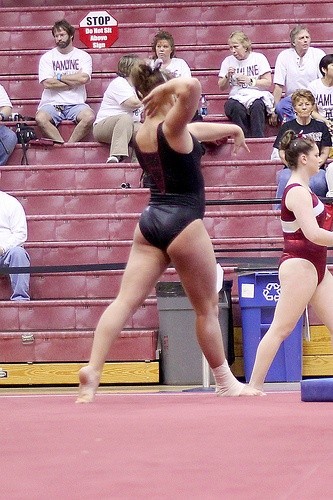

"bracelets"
[56,73,61,81]
[0,112,4,121]
[225,73,232,83]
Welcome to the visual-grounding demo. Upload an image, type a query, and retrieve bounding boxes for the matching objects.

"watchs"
[248,78,253,86]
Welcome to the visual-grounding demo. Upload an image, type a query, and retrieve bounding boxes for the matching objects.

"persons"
[144,31,203,123]
[247,130,333,391]
[326,161,333,198]
[218,29,271,139]
[33,19,96,142]
[273,26,327,125]
[0,169,30,302]
[306,53,332,149]
[92,54,144,163]
[0,83,17,166]
[270,89,332,220]
[74,59,261,404]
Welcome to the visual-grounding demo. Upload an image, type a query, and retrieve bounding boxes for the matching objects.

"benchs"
[0,0,333,364]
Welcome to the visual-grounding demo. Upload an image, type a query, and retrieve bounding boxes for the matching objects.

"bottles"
[199,93,208,117]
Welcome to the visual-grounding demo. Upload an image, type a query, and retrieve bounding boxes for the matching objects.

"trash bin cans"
[155,280,235,387]
[238,272,304,384]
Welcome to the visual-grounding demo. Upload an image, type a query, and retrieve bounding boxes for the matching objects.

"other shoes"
[106,156,119,163]
